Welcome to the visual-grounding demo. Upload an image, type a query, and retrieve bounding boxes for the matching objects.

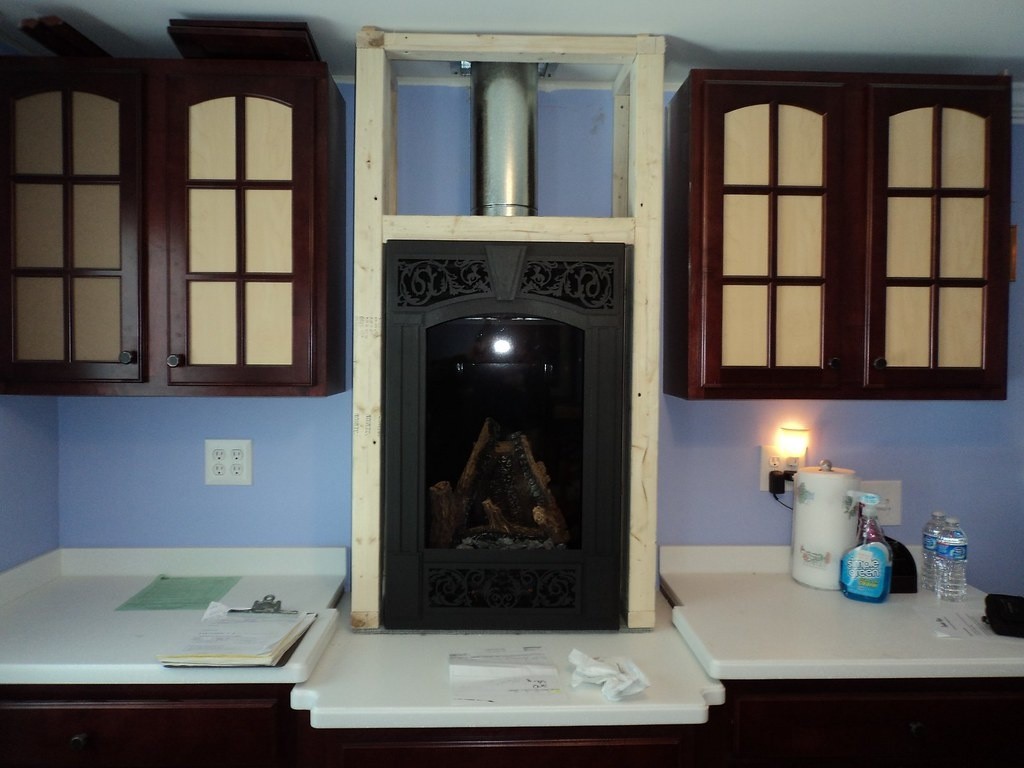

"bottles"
[921,511,946,591]
[938,518,967,601]
[935,526,945,598]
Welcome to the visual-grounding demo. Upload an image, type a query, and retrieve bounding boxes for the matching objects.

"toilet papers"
[790,468,860,592]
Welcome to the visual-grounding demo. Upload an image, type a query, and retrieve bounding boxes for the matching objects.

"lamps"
[775,423,809,456]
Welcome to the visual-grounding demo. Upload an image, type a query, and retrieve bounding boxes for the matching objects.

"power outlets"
[860,478,900,526]
[760,444,808,492]
[204,439,252,486]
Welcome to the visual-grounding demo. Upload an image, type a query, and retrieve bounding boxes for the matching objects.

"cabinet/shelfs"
[0,678,1024,768]
[664,70,1011,401]
[0,14,347,397]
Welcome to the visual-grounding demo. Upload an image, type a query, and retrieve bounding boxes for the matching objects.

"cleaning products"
[839,493,891,605]
